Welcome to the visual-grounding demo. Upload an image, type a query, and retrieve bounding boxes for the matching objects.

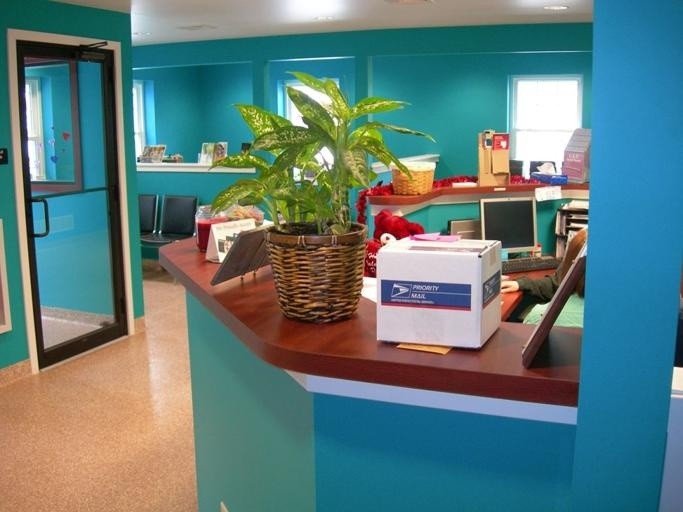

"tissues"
[531,162,569,184]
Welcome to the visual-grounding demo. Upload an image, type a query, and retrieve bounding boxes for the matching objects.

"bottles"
[195,206,226,253]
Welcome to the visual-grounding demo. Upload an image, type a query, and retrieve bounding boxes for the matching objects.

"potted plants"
[207,68,436,325]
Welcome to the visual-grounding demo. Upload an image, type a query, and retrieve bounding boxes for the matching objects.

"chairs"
[138,194,201,286]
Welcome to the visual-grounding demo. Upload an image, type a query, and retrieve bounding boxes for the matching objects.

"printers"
[554,200,588,259]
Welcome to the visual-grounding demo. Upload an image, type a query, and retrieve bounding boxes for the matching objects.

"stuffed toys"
[362,210,423,277]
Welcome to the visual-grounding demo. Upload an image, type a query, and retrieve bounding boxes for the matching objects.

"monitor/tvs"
[479,197,538,261]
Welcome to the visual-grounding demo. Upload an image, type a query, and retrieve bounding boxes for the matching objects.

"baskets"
[391,162,436,195]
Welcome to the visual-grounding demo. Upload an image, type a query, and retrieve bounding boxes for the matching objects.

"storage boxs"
[376,234,503,349]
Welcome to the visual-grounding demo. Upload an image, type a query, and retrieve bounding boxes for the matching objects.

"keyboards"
[501,257,561,275]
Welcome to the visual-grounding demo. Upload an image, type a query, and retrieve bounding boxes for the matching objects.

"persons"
[500,229,585,302]
